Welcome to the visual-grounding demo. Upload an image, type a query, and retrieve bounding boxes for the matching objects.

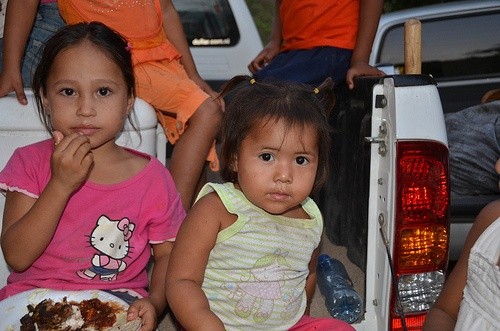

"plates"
[0,289,141,331]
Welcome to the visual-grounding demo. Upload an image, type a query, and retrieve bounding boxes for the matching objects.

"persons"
[248,0,387,91]
[165,76,357,331]
[0,0,68,87]
[423,157,500,331]
[0,21,188,331]
[0,0,226,213]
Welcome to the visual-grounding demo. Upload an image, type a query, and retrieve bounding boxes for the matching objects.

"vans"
[368,0,499,271]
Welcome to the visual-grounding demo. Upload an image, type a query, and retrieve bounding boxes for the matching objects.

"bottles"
[315,253,363,324]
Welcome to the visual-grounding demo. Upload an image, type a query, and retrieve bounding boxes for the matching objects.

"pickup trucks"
[0,0,453,331]
